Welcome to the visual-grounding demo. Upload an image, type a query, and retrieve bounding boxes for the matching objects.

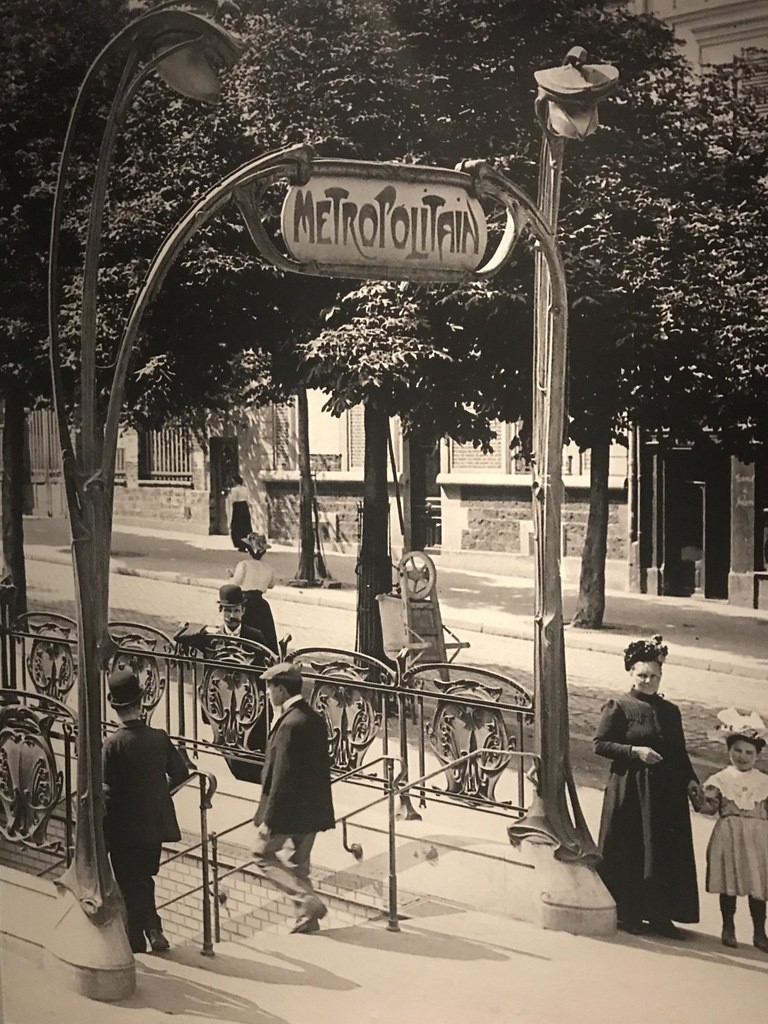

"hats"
[107,671,144,708]
[259,662,302,682]
[707,707,767,753]
[241,533,271,554]
[219,584,247,606]
[624,634,668,671]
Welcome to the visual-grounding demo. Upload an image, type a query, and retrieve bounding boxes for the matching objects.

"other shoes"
[290,904,327,933]
[147,927,169,951]
[649,918,685,940]
[753,935,768,952]
[722,926,737,947]
[616,916,652,935]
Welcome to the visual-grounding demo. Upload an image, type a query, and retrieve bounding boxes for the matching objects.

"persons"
[102,671,190,951]
[592,635,705,937]
[694,735,768,952]
[201,585,273,783]
[254,663,337,933]
[226,476,253,552]
[225,531,278,654]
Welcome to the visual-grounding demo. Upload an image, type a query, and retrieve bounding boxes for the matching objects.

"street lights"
[47,2,256,1001]
[518,45,623,944]
